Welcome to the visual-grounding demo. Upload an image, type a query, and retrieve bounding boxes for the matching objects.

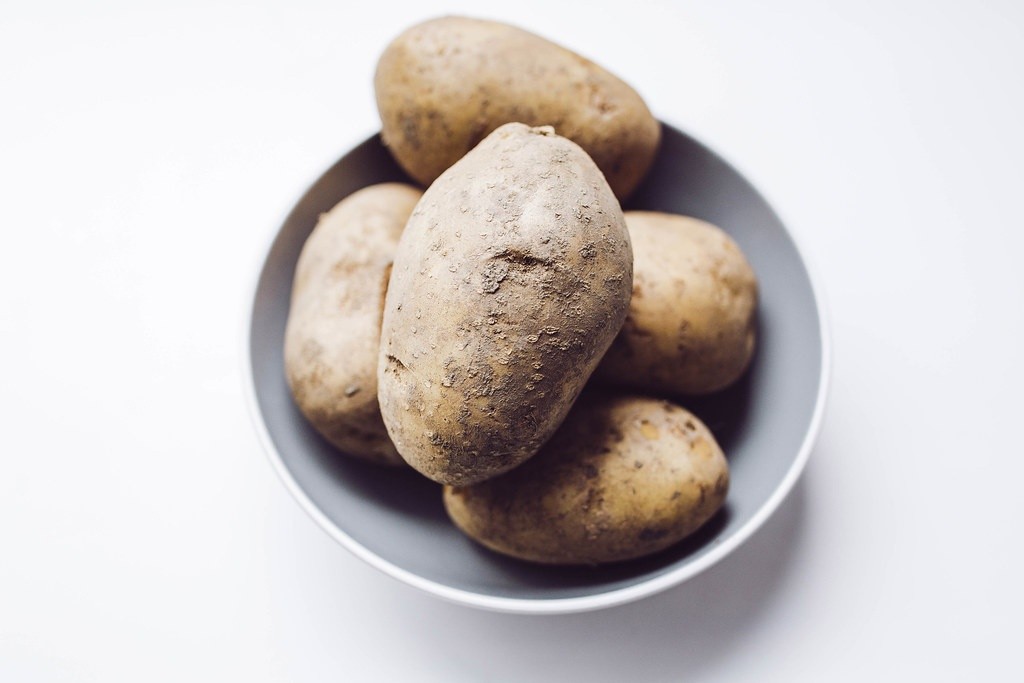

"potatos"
[284,14,758,562]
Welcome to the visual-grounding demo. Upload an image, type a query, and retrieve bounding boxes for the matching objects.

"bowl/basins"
[243,117,831,616]
